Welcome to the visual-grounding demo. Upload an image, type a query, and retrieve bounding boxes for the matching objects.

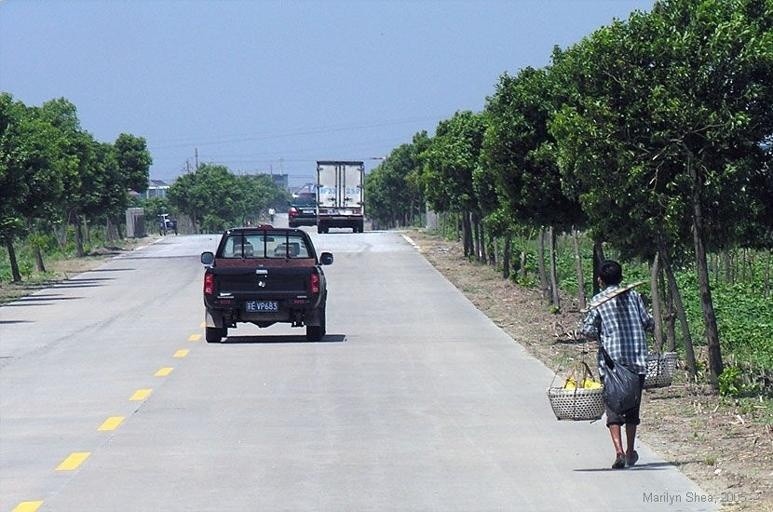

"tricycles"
[158,214,178,236]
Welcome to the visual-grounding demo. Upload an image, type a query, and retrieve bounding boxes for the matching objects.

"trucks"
[316,159,365,234]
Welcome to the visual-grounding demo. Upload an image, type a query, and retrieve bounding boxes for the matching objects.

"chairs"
[234,242,300,258]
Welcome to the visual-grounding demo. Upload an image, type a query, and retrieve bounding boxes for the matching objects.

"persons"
[582,260,652,468]
[157,201,171,229]
[269,205,275,224]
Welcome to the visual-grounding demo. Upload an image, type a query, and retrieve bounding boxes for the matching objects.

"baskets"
[642,337,678,392]
[546,361,605,422]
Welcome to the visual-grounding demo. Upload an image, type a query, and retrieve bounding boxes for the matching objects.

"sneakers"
[611,450,638,469]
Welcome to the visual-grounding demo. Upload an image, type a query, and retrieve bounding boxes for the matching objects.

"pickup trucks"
[201,225,333,343]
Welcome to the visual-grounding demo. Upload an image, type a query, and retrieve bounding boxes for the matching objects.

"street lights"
[373,157,399,228]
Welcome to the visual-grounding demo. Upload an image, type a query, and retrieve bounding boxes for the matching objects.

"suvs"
[287,196,317,227]
